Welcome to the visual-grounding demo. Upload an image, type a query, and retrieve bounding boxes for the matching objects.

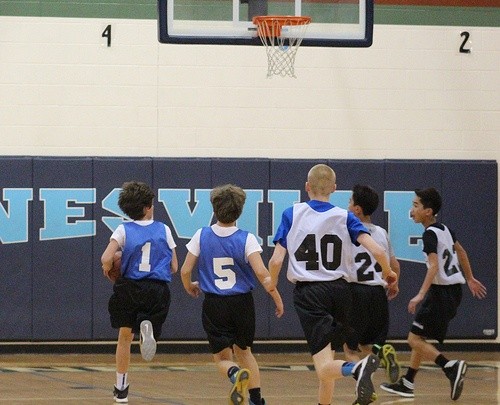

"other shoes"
[229,369,250,405]
[249,394,266,405]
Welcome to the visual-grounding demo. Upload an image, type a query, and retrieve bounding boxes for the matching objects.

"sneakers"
[352,393,378,405]
[112,385,130,403]
[350,353,380,405]
[443,359,467,400]
[381,344,400,385]
[380,381,414,398]
[139,320,156,361]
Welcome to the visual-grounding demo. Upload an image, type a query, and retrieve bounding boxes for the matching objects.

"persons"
[262,164,398,405]
[181,184,284,405]
[349,183,401,405]
[381,187,487,401]
[101,180,179,403]
